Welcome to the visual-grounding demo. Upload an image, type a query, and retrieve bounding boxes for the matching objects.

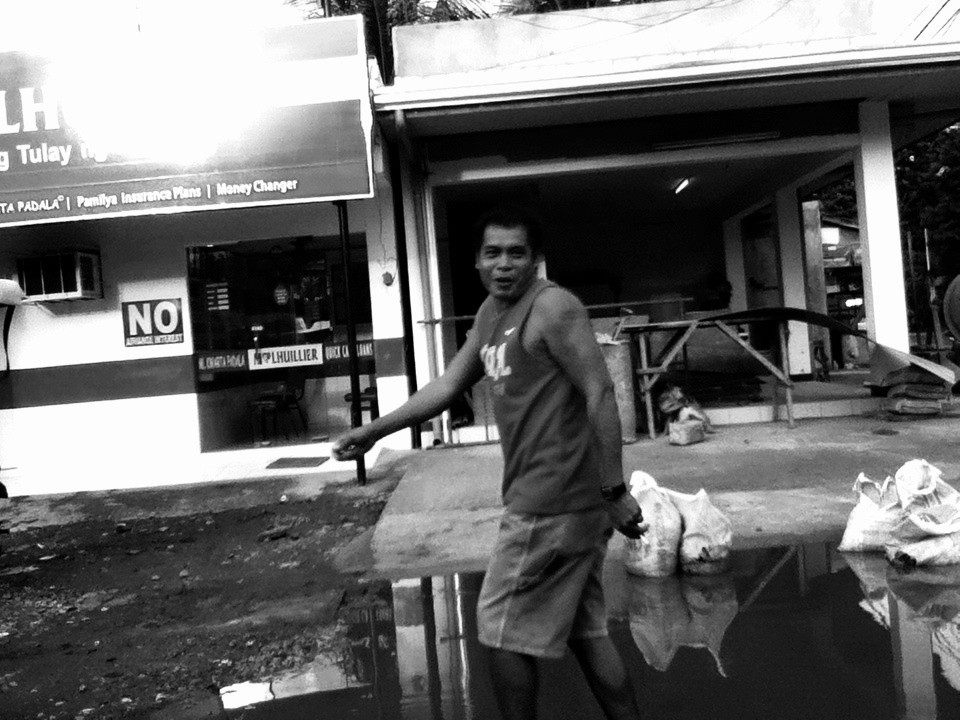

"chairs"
[248,378,308,443]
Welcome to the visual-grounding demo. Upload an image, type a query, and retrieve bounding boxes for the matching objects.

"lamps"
[674,174,694,195]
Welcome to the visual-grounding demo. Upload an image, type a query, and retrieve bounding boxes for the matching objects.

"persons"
[329,208,651,720]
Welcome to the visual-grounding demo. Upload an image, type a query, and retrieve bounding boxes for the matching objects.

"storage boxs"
[668,420,705,445]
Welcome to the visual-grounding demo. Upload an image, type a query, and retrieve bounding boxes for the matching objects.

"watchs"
[599,481,627,501]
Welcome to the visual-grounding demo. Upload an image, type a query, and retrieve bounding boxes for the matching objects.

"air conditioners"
[12,250,103,303]
